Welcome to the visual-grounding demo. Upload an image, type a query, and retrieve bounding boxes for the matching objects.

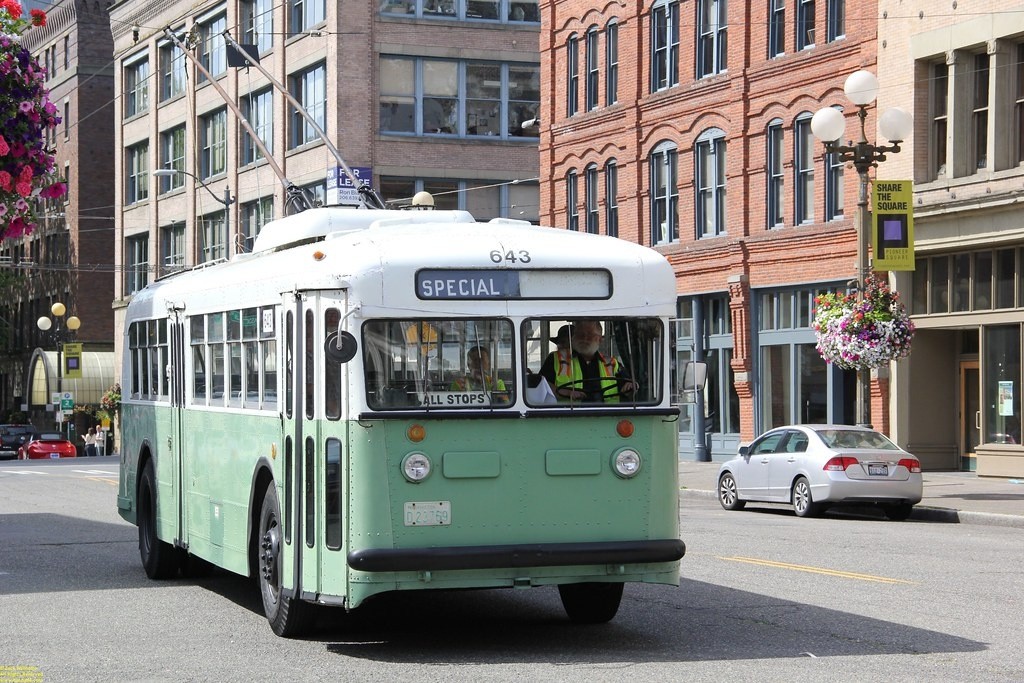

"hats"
[549,325,575,343]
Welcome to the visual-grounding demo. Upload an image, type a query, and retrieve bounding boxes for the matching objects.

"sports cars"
[18,430,76,460]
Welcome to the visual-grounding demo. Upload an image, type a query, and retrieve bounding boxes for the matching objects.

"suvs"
[0,424,38,457]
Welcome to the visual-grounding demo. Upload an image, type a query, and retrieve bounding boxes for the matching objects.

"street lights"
[809,70,913,428]
[37,302,81,433]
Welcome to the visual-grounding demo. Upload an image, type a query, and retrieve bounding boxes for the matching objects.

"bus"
[112,30,685,638]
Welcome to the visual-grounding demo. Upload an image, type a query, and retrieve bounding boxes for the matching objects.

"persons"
[95,425,105,456]
[548,324,577,352]
[80,427,96,457]
[538,319,640,404]
[450,346,510,405]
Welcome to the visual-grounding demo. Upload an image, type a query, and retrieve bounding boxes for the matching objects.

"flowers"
[101,383,121,416]
[0,0,72,248]
[810,275,916,371]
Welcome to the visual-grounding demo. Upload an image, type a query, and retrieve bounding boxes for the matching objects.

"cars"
[718,424,923,518]
